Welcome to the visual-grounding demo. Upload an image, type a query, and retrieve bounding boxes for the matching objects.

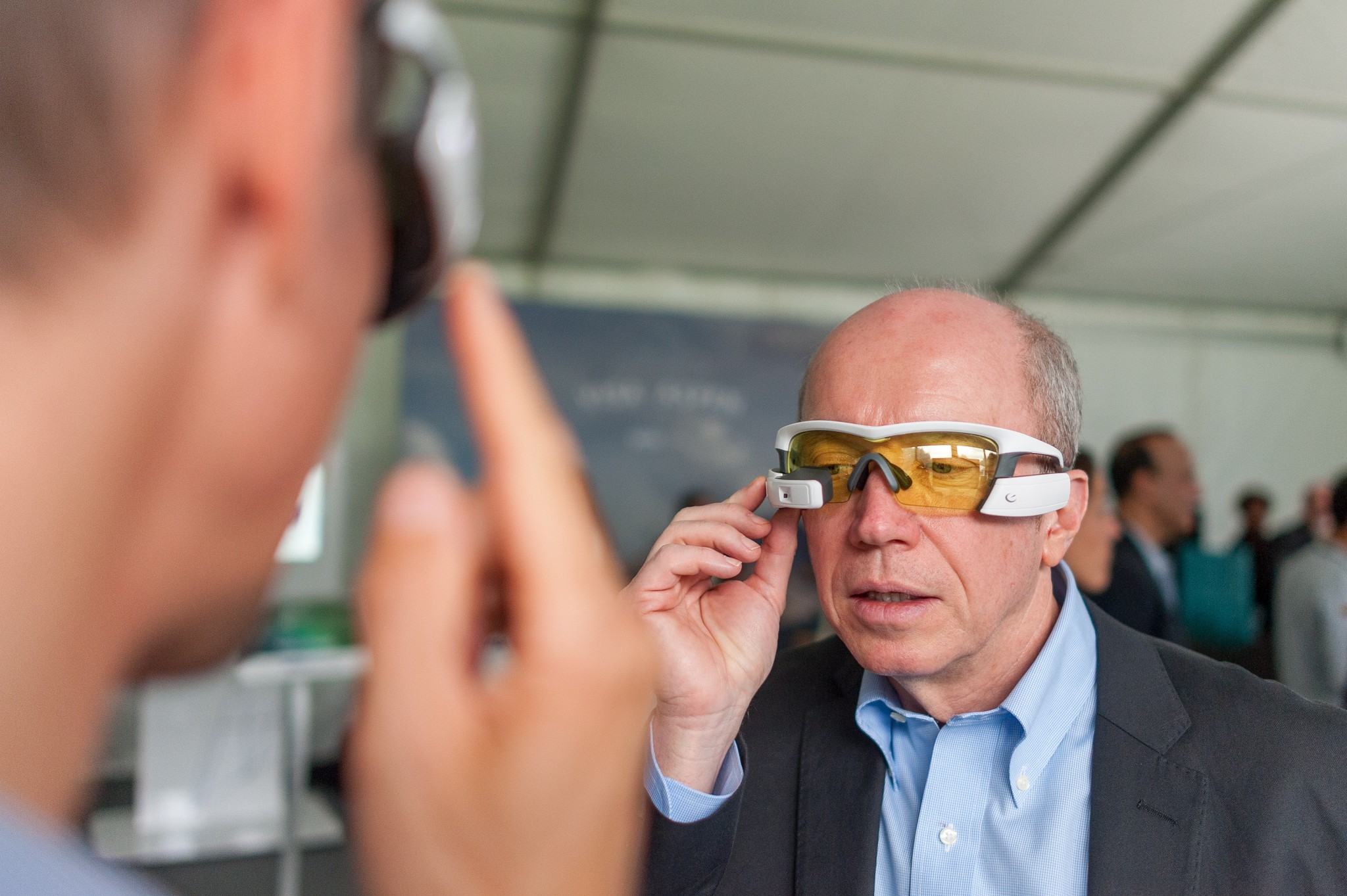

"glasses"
[764,414,1069,517]
[357,1,482,324]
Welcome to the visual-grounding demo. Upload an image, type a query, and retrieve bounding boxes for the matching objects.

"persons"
[1062,421,1347,709]
[617,274,1347,896]
[676,486,713,506]
[0,0,665,896]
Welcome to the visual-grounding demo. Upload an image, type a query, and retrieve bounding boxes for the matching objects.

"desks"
[235,642,518,896]
